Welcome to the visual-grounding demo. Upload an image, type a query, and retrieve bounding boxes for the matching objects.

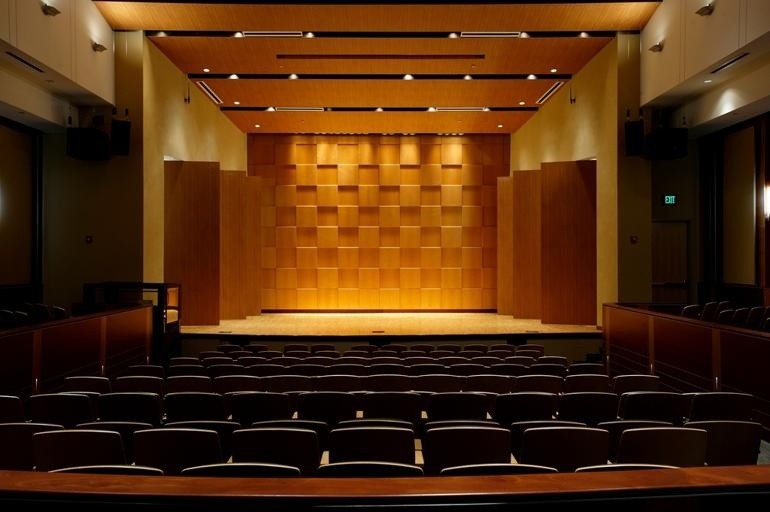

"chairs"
[3,301,64,326]
[0,344,762,480]
[676,298,769,333]
